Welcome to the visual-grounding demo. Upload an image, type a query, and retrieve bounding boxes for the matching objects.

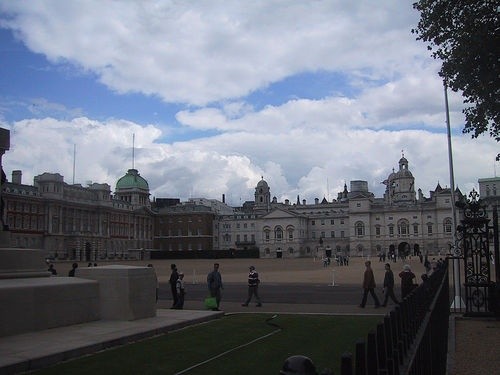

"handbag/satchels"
[204,297,217,309]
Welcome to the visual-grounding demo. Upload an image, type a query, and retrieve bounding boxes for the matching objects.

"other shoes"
[381,304,387,307]
[256,303,261,307]
[375,305,378,308]
[242,304,248,306]
[360,302,365,307]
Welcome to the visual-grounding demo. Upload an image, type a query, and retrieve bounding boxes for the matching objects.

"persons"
[359,260,381,309]
[47,263,100,277]
[424,257,443,274]
[147,264,159,305]
[207,263,224,311]
[376,250,423,263]
[398,265,416,300]
[167,264,185,310]
[322,255,350,269]
[241,266,263,307]
[381,263,400,307]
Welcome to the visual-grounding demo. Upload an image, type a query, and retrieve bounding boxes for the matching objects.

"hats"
[248,266,255,270]
[404,265,409,272]
